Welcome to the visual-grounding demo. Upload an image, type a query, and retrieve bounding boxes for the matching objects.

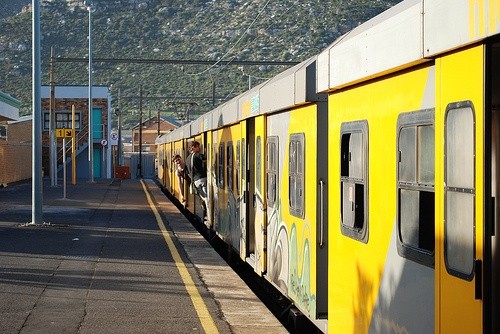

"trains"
[152,2,499,334]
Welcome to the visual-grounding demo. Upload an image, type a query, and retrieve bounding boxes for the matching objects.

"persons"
[184,141,208,221]
[172,154,205,207]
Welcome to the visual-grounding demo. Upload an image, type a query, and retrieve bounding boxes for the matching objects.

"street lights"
[83,1,95,184]
